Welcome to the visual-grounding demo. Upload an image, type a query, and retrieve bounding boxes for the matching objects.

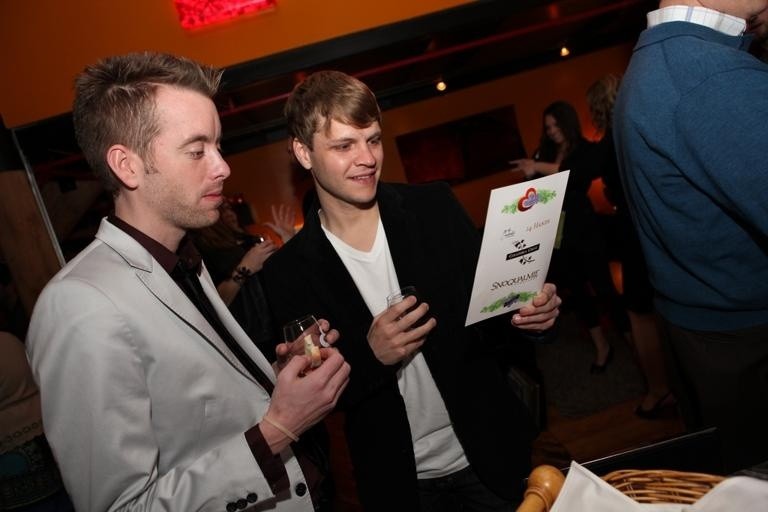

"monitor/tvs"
[392,105,529,188]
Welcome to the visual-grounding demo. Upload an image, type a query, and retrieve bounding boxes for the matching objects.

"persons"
[509,103,613,376]
[587,66,695,418]
[259,72,563,512]
[22,51,353,512]
[610,0,768,477]
[193,161,319,362]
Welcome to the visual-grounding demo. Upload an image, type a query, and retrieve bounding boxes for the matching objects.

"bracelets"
[266,415,299,448]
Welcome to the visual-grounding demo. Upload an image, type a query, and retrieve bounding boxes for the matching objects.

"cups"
[384,285,426,343]
[282,313,328,371]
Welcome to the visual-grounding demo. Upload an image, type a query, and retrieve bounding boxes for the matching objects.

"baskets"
[600,469,728,504]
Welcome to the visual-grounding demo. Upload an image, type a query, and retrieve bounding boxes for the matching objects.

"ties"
[171,261,307,463]
